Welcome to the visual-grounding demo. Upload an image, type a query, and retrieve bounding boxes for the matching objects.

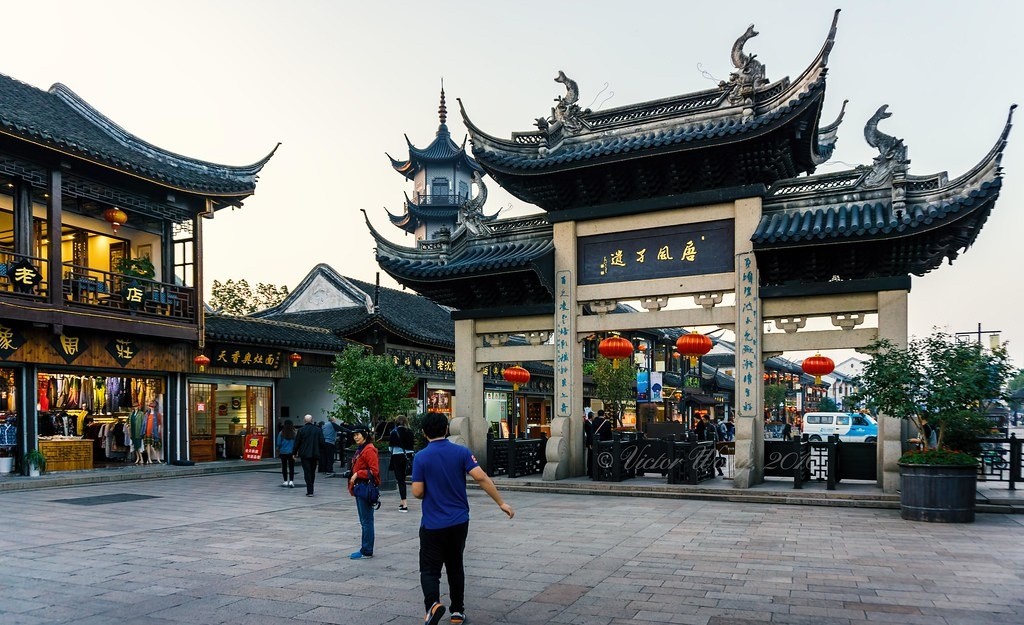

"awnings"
[689,394,720,406]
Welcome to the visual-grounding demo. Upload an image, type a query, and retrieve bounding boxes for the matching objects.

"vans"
[802,413,878,442]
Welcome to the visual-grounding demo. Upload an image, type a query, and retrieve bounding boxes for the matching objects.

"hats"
[351,425,371,434]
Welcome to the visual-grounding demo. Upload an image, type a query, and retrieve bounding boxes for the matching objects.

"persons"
[143,403,162,463]
[389,415,414,513]
[582,410,613,480]
[129,402,145,464]
[691,412,803,476]
[82,402,92,438]
[896,410,937,494]
[348,426,380,560]
[411,412,515,625]
[277,413,397,497]
[997,416,1009,438]
[1013,410,1017,426]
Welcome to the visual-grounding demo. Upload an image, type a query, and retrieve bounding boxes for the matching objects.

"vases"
[897,461,979,523]
[0,457,13,474]
[345,448,392,490]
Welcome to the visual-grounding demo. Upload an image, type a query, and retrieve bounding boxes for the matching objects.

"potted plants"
[229,416,243,435]
[24,448,47,477]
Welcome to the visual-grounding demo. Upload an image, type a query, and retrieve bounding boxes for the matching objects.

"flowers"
[899,446,980,466]
[348,438,387,451]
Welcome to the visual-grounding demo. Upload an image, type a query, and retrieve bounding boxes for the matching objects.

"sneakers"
[349,551,372,559]
[398,504,404,508]
[288,481,294,488]
[283,481,288,486]
[399,507,408,513]
[424,602,446,625]
[450,612,466,625]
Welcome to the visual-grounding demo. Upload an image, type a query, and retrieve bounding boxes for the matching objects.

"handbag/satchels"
[405,457,412,476]
[352,467,382,510]
[714,452,726,467]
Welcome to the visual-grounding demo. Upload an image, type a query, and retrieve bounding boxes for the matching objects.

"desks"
[145,292,180,316]
[0,263,16,292]
[62,279,108,304]
[216,433,245,459]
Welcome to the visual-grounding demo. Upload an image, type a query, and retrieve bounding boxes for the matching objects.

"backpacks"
[716,423,723,436]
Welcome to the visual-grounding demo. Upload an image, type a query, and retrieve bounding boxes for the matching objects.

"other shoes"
[718,473,724,476]
[326,471,335,474]
[306,493,314,497]
[896,489,901,494]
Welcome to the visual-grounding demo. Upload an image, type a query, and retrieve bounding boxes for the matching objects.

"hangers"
[45,410,78,419]
[47,376,106,381]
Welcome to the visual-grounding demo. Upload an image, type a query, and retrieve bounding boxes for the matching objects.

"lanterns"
[504,365,531,390]
[802,354,835,385]
[763,373,827,392]
[105,207,128,234]
[598,335,634,370]
[676,331,713,368]
